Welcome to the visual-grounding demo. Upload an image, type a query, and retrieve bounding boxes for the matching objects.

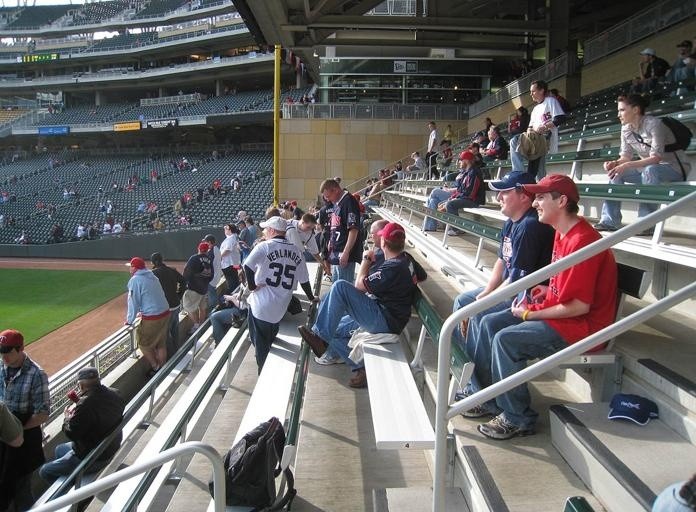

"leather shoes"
[297,325,327,358]
[348,368,366,387]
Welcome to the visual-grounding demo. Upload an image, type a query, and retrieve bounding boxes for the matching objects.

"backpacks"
[209,417,294,512]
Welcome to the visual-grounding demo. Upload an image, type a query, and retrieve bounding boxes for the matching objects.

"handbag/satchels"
[232,307,248,329]
[658,117,693,151]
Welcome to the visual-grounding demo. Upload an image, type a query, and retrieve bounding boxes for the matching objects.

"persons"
[38,364,123,496]
[628,47,671,99]
[0,327,51,510]
[462,173,619,440]
[0,400,25,511]
[298,218,427,391]
[588,92,689,230]
[448,171,555,404]
[2,0,573,241]
[121,176,367,378]
[649,38,695,101]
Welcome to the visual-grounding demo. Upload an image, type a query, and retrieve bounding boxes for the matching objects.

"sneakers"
[187,328,198,335]
[323,274,332,281]
[454,383,474,401]
[314,351,346,365]
[636,226,655,236]
[146,366,162,378]
[447,229,465,236]
[592,223,618,231]
[461,402,503,417]
[477,412,535,440]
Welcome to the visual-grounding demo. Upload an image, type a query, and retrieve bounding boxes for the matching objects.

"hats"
[607,393,659,426]
[0,329,23,353]
[126,257,145,269]
[198,234,215,251]
[641,48,656,56]
[522,175,579,203]
[260,216,287,232]
[78,368,99,380]
[456,131,483,160]
[236,220,245,225]
[488,171,537,191]
[377,222,405,242]
[676,40,692,47]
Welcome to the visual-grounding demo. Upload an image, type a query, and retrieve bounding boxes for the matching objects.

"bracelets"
[614,159,620,166]
